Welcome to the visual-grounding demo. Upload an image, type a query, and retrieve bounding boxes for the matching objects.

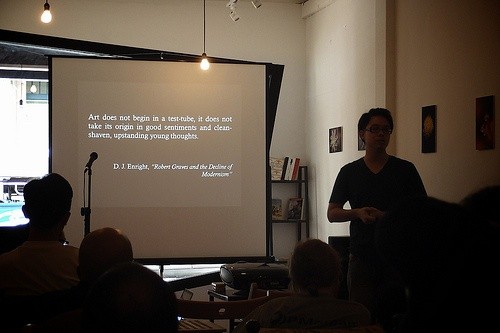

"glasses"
[364,126,392,134]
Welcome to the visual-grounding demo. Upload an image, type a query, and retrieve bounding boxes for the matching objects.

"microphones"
[84,151,98,173]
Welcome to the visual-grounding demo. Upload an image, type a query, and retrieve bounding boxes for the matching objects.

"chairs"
[177,295,270,333]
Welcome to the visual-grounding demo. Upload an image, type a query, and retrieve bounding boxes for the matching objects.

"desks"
[208,288,291,333]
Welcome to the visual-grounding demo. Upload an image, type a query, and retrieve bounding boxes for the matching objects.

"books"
[287,198,304,220]
[272,199,283,219]
[272,157,300,180]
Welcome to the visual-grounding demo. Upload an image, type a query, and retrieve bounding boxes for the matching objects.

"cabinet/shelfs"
[268,165,309,262]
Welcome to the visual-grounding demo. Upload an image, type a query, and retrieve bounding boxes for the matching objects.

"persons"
[0,173,79,299]
[233,239,371,333]
[328,108,427,324]
[36,228,133,333]
[374,186,500,333]
[81,262,179,333]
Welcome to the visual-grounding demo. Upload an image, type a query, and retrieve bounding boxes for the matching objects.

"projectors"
[218,261,291,291]
